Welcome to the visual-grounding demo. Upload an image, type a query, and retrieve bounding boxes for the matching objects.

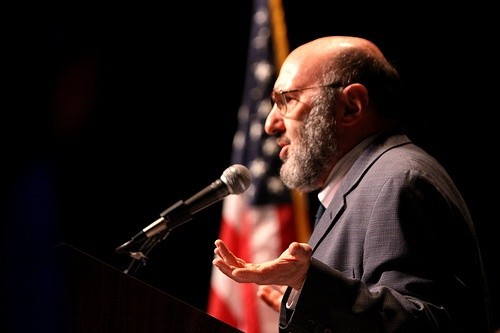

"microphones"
[114,164,253,255]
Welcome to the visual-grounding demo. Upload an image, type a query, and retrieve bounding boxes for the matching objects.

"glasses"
[271,81,349,116]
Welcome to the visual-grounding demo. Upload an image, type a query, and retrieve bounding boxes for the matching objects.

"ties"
[310,200,331,241]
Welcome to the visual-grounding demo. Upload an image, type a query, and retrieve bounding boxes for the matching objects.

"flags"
[206,0,302,333]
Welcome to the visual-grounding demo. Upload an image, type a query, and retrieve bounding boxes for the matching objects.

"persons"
[211,36,487,333]
